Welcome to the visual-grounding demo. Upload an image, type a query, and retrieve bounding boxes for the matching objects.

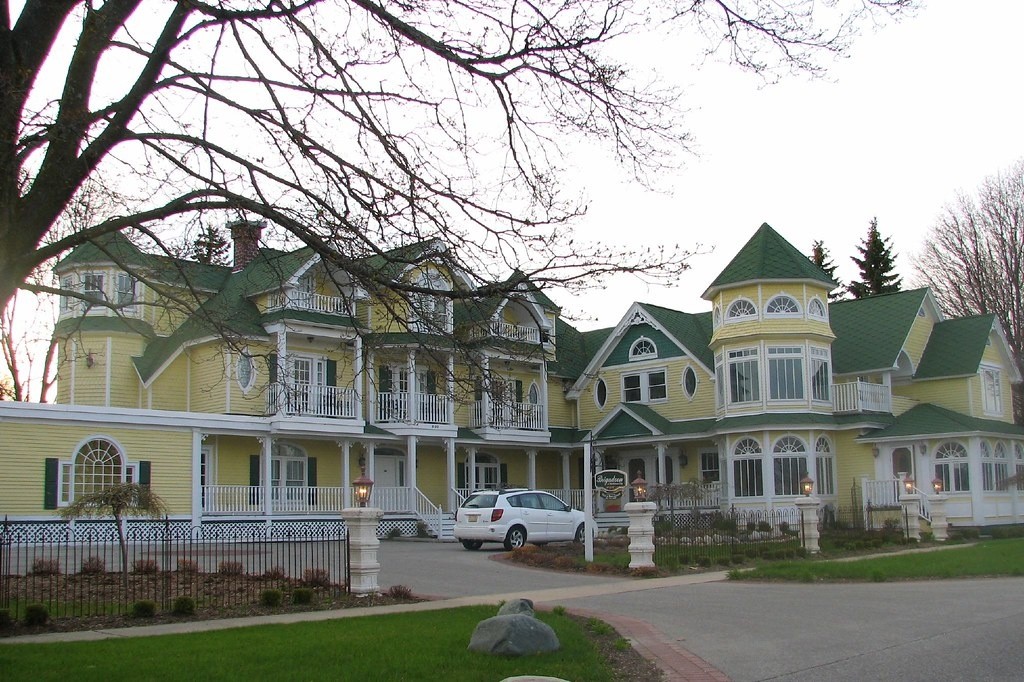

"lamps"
[902,473,915,494]
[918,440,926,455]
[86,352,93,368]
[799,471,814,499]
[358,453,365,468]
[931,473,942,495]
[351,464,375,508]
[871,443,879,459]
[678,448,687,468]
[630,470,649,502]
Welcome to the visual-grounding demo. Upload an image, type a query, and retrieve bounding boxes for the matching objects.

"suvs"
[453,487,598,552]
